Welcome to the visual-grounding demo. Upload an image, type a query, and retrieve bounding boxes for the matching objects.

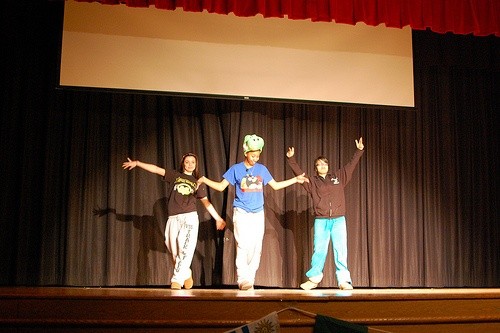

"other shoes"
[184,270,194,289]
[342,282,353,290]
[171,282,181,290]
[300,280,318,290]
[240,282,252,291]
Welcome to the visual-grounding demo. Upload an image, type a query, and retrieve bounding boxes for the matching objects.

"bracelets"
[136,160,139,166]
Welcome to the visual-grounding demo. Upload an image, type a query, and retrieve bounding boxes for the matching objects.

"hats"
[242,133,265,155]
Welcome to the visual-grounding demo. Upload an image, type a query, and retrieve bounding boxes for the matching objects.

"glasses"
[315,163,328,166]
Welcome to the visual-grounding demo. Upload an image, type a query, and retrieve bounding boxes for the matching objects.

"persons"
[197,132,309,290]
[285,135,366,291]
[122,151,226,290]
